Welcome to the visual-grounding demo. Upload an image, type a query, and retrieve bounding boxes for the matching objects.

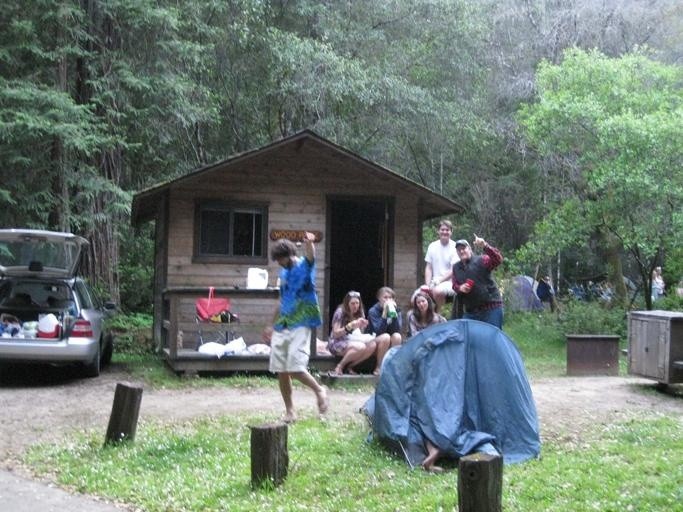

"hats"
[454,238,469,248]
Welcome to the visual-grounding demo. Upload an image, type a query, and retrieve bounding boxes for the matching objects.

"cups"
[465,278,475,289]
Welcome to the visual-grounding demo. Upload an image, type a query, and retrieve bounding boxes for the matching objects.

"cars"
[0,227,112,375]
[566,277,641,305]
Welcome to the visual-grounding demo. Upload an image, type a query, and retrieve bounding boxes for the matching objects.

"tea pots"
[246,268,269,289]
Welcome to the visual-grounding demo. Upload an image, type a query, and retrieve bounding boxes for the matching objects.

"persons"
[647,270,661,307]
[420,439,447,475]
[366,286,403,377]
[537,276,554,314]
[328,291,377,376]
[406,285,448,341]
[450,233,505,331]
[423,219,462,312]
[655,266,666,299]
[270,231,330,423]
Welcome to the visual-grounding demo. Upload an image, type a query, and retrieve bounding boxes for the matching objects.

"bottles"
[386,301,397,319]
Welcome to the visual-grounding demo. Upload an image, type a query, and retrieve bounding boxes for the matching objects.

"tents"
[508,274,551,313]
[359,316,542,470]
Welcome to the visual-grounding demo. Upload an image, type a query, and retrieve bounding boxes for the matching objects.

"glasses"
[347,291,362,298]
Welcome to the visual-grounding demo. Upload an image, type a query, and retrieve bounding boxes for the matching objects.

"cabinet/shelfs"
[623,307,683,385]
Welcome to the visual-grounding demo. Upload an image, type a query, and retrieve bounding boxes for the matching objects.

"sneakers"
[314,383,333,414]
[335,367,381,376]
[282,409,299,424]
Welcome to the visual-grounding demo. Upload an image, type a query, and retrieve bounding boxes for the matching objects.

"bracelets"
[483,244,487,250]
[344,322,354,336]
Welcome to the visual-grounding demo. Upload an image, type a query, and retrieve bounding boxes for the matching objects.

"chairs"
[192,295,235,351]
